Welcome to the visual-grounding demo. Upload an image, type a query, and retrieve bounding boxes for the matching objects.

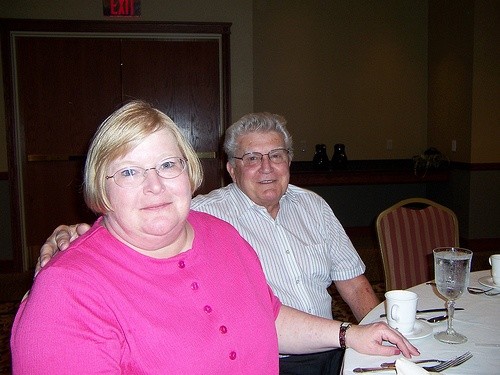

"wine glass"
[433,246,473,344]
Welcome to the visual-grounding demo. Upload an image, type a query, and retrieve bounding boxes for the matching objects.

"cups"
[385,290,418,335]
[488,253,500,287]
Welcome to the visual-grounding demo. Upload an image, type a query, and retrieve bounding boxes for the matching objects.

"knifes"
[380,307,464,318]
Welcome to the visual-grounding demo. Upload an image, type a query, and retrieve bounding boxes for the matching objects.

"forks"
[426,281,495,294]
[353,358,459,373]
[381,351,473,368]
[467,287,500,296]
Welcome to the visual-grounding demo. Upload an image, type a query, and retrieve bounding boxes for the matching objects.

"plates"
[479,274,500,290]
[398,318,432,341]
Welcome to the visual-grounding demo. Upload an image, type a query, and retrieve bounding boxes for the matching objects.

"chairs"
[377,198,459,291]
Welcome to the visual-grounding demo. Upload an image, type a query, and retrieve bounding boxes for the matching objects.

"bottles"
[330,143,348,174]
[312,144,329,170]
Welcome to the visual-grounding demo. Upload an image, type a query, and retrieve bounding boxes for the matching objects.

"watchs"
[339,322,351,348]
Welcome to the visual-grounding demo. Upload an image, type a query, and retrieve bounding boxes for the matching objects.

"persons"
[10,100,421,375]
[35,111,381,375]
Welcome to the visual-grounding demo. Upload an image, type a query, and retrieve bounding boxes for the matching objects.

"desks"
[340,270,500,375]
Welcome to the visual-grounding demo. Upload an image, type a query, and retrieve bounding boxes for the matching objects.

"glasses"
[105,157,188,189]
[232,148,289,167]
